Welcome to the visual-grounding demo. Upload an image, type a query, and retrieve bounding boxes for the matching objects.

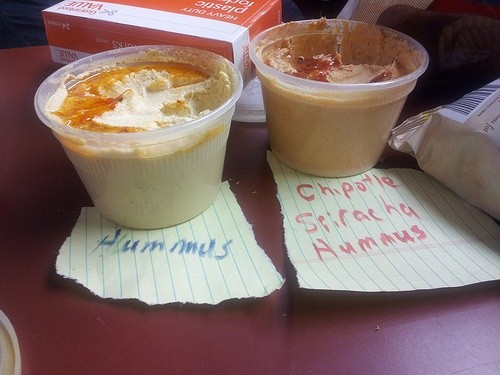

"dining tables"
[0,43,500,375]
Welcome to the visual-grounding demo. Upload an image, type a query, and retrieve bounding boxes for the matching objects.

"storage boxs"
[40,0,284,90]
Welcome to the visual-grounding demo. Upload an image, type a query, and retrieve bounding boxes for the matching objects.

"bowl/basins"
[32,43,243,230]
[248,16,428,180]
